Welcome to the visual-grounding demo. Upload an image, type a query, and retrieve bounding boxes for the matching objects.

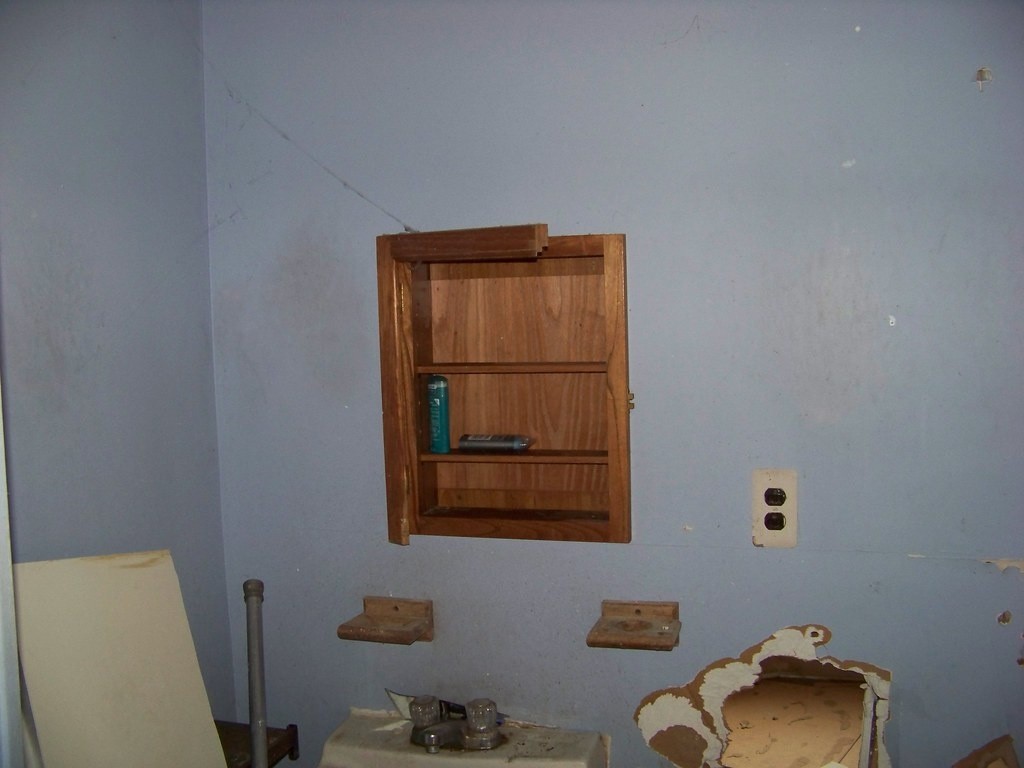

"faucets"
[419,722,466,753]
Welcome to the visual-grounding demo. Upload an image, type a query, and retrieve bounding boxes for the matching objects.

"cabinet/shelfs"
[377,223,632,547]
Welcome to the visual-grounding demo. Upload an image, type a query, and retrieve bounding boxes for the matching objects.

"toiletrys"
[424,375,450,457]
[457,432,534,454]
